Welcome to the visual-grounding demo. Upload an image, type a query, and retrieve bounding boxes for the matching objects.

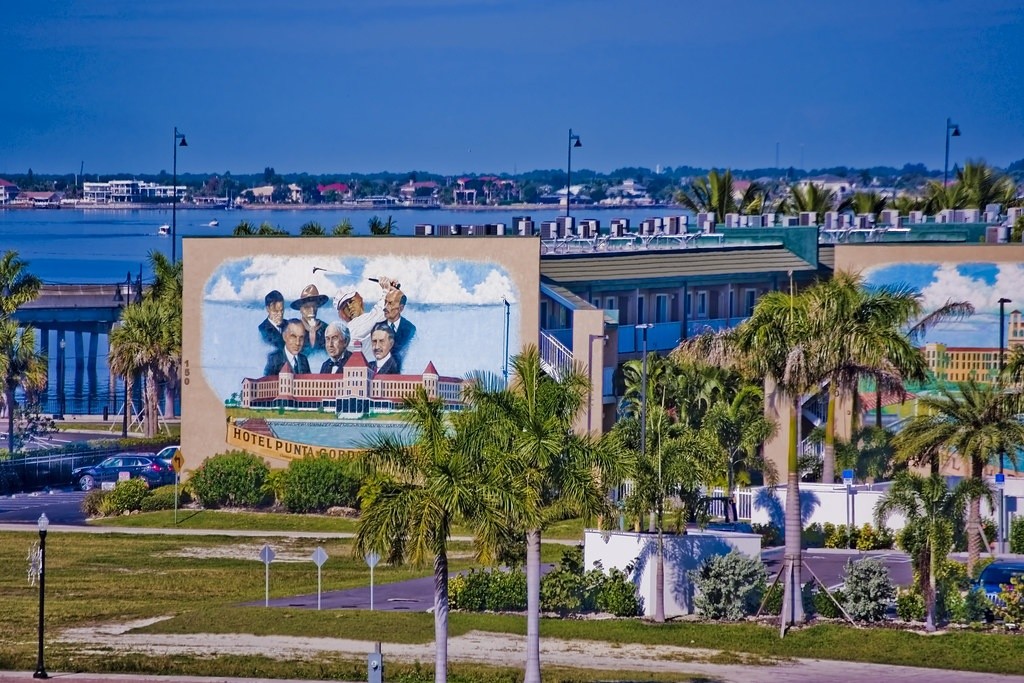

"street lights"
[635,323,655,456]
[59,338,67,421]
[171,125,188,268]
[943,117,962,190]
[995,297,1013,555]
[566,128,584,218]
[33,511,50,678]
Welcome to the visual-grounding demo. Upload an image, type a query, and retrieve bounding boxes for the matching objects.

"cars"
[72,444,181,492]
[969,557,1024,624]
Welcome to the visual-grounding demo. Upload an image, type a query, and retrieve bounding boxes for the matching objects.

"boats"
[159,224,172,235]
[209,220,219,226]
